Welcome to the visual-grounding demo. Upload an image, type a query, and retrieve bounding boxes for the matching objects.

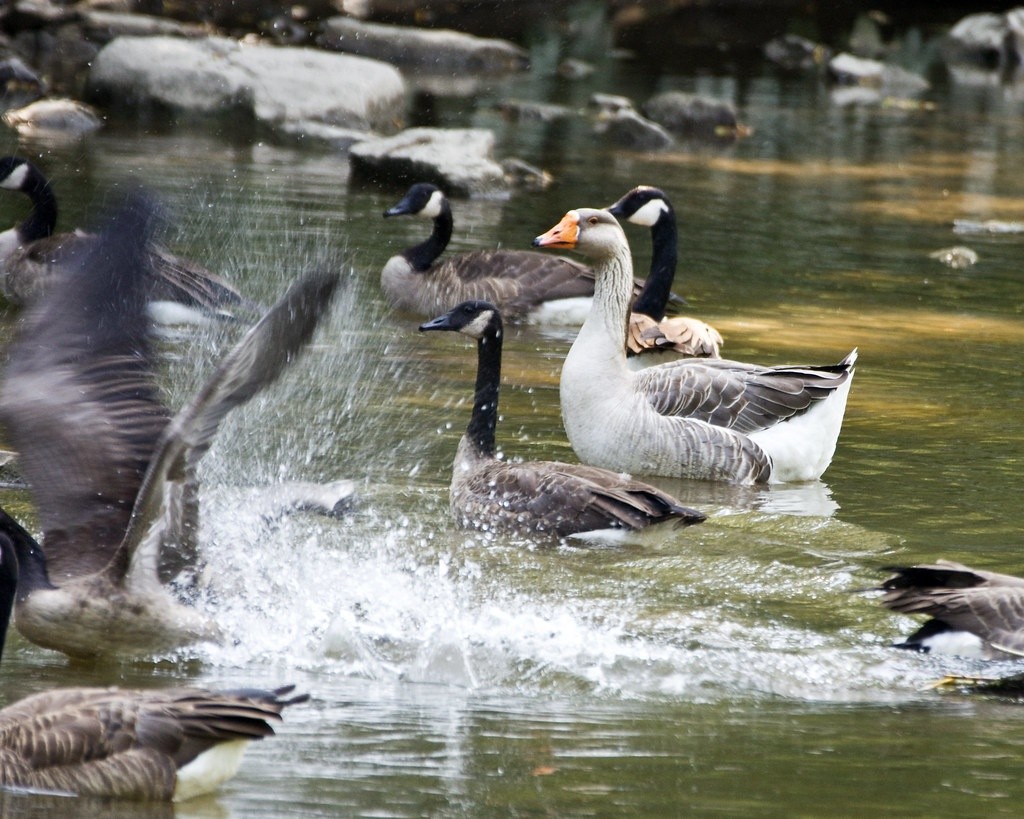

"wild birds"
[0,193,342,661]
[597,186,723,372]
[0,155,242,332]
[530,207,860,486]
[379,184,691,327]
[0,533,309,803]
[869,556,1024,661]
[416,301,709,549]
